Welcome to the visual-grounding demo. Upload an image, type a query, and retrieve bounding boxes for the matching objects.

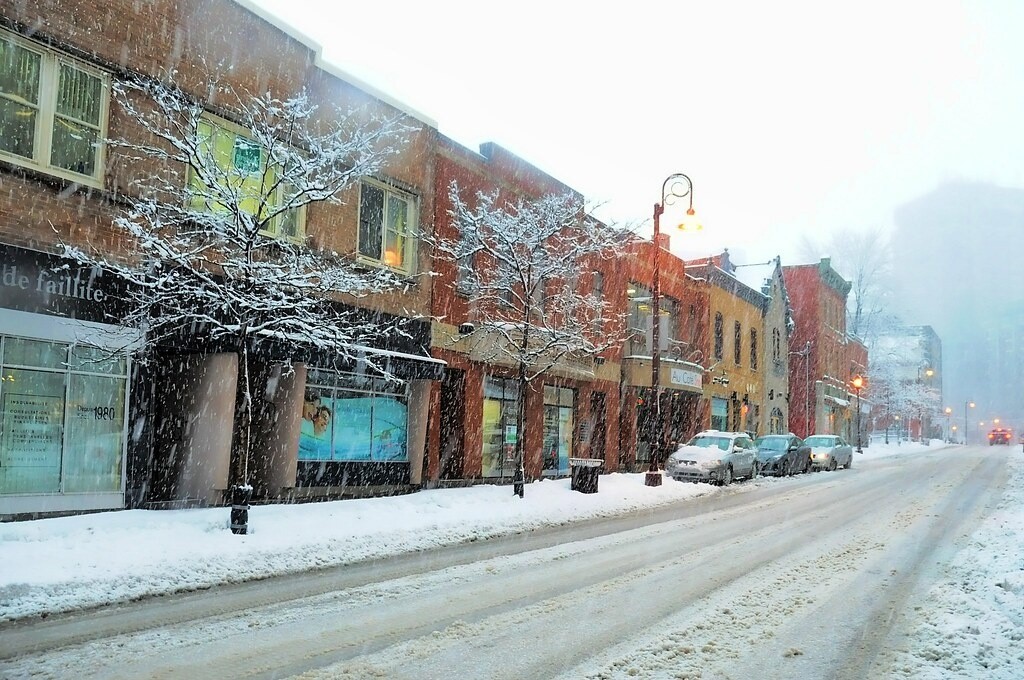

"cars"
[662,430,761,487]
[987,428,1012,446]
[803,434,854,473]
[753,433,813,477]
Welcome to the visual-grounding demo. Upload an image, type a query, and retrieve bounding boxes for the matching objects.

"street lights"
[945,407,953,442]
[916,356,934,442]
[850,373,865,455]
[963,400,976,446]
[644,172,703,486]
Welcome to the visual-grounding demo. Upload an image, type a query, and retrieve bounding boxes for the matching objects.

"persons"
[300,388,330,437]
[507,426,516,439]
